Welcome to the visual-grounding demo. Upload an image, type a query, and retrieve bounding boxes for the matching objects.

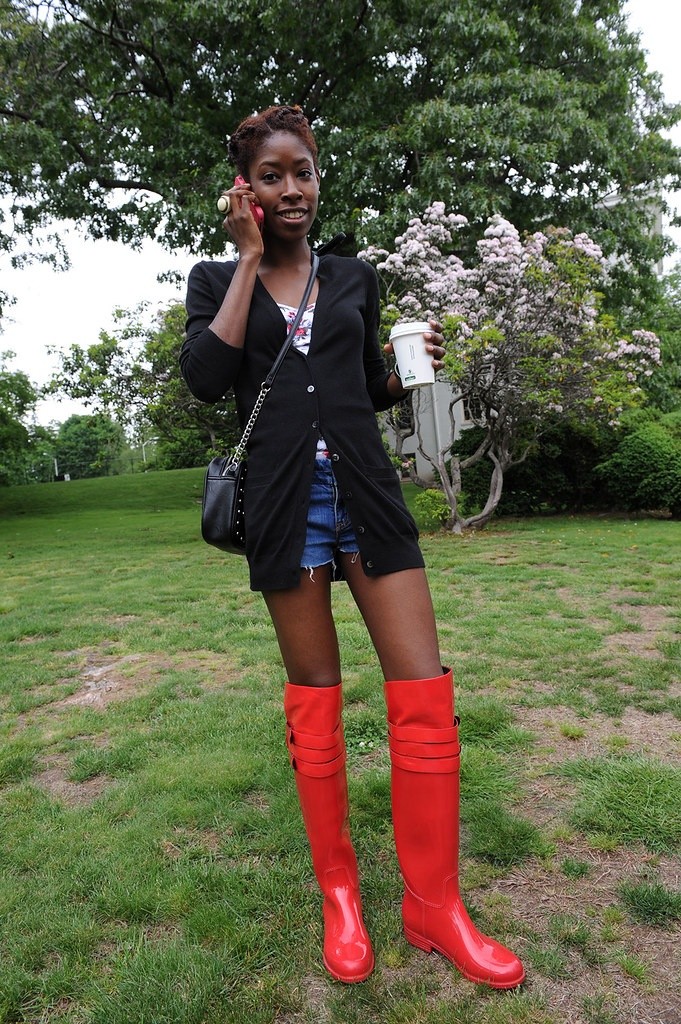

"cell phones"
[234,176,263,236]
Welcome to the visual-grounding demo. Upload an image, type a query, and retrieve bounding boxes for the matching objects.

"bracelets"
[393,362,401,381]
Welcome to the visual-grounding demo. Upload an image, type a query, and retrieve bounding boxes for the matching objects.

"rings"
[217,196,231,214]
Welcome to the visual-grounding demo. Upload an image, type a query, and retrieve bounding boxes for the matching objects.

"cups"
[389,322,435,390]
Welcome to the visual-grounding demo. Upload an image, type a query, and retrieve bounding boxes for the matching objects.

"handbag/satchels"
[201,456,247,555]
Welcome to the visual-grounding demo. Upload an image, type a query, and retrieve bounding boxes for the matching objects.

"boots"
[385,665,526,989]
[283,682,374,983]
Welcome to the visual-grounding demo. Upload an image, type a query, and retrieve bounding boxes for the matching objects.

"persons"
[180,105,525,987]
